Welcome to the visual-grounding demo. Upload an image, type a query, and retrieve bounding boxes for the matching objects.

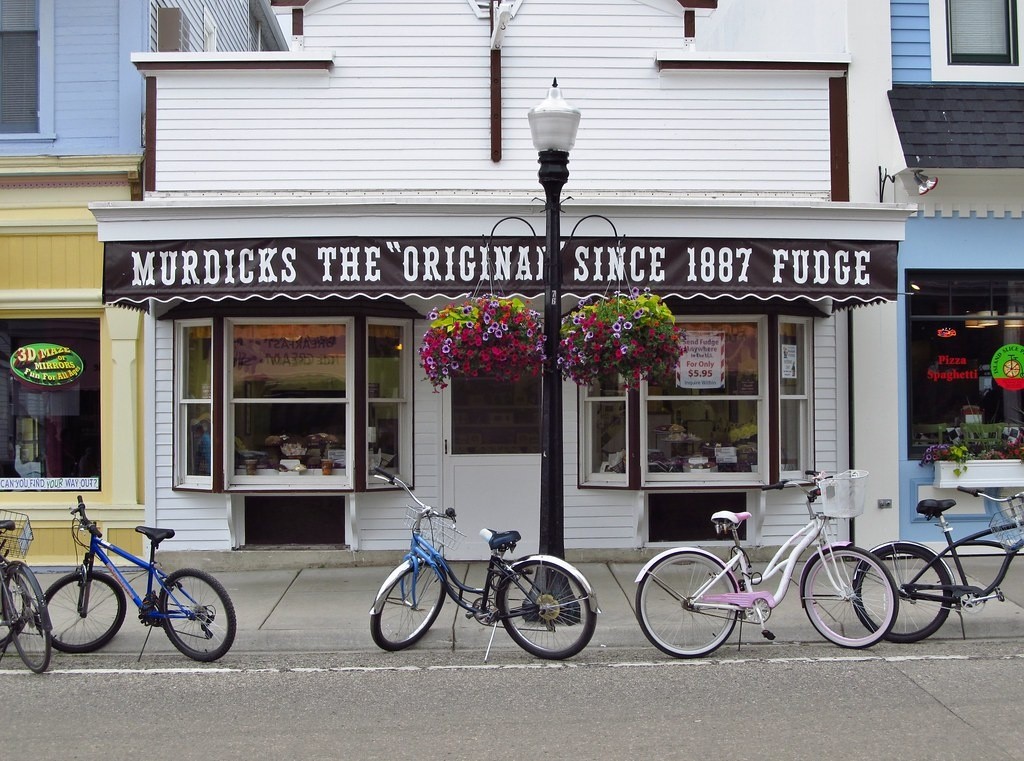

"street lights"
[520,77,583,628]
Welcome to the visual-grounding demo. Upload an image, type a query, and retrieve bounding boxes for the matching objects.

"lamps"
[912,170,938,196]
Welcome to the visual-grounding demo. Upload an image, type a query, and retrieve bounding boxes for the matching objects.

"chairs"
[958,422,1002,446]
[911,424,948,446]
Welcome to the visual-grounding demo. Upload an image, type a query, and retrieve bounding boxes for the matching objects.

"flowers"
[418,290,546,395]
[961,404,981,414]
[559,286,685,395]
[729,425,757,446]
[919,426,1024,478]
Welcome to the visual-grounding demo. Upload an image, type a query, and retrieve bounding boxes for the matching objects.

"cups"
[320,459,334,476]
[244,460,258,475]
[201,384,211,399]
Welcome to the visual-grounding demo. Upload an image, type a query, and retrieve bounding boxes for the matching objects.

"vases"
[965,413,982,424]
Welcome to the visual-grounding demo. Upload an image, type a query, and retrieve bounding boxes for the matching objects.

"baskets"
[819,469,870,518]
[0,507,35,559]
[403,503,469,555]
[989,505,1024,553]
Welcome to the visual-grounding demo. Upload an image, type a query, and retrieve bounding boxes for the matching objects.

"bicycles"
[0,509,53,674]
[633,470,900,661]
[370,465,602,662]
[850,486,1024,645]
[32,494,238,663]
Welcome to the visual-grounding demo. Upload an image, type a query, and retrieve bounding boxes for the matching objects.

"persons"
[193,421,211,475]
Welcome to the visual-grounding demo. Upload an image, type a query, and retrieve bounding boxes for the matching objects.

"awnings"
[101,237,903,317]
